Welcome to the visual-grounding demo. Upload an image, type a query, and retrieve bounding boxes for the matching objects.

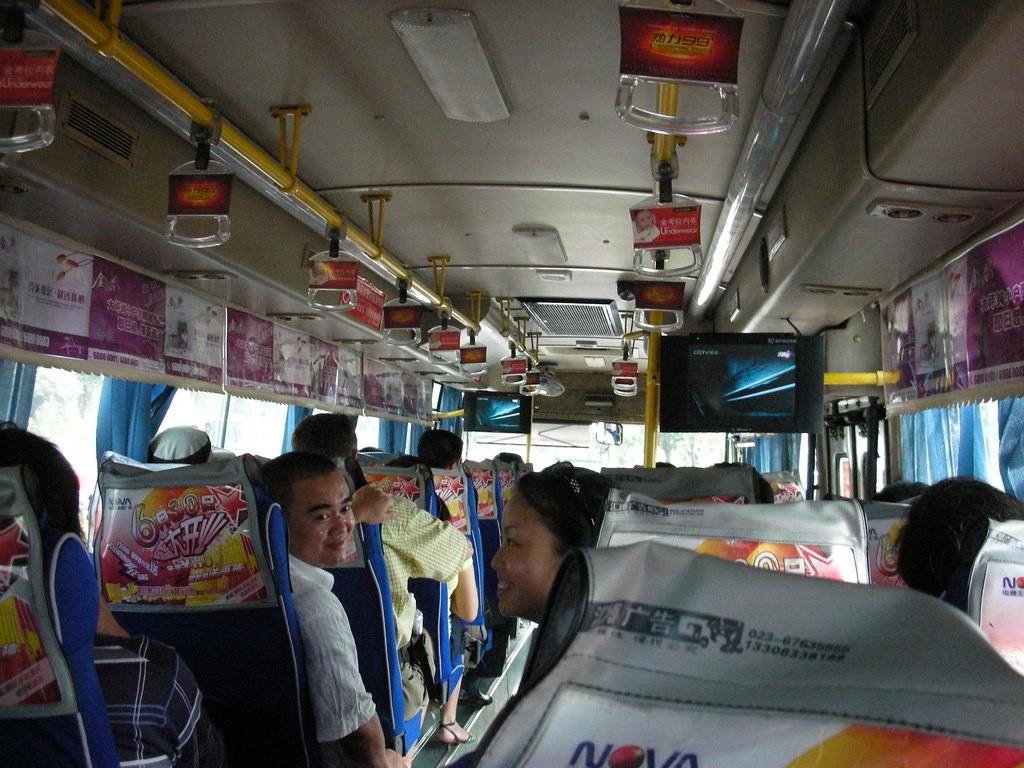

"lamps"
[388,5,514,123]
[512,226,568,265]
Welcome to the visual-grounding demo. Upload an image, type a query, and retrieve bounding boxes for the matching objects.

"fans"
[536,362,565,397]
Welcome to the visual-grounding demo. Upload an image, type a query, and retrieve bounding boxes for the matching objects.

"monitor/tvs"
[462,391,532,434]
[658,333,825,435]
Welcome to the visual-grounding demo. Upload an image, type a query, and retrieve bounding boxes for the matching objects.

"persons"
[261,450,407,768]
[291,414,474,720]
[440,462,620,768]
[386,454,479,751]
[865,476,1024,613]
[417,430,493,708]
[0,419,224,768]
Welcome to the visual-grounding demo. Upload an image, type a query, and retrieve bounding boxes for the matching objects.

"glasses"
[548,461,597,528]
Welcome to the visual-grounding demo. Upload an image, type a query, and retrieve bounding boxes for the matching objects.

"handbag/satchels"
[398,634,437,700]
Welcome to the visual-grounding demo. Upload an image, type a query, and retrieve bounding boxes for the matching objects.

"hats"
[147,425,212,467]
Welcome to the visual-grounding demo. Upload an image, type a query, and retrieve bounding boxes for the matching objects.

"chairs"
[0,449,1024,768]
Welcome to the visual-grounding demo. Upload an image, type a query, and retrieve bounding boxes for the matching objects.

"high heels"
[438,720,475,751]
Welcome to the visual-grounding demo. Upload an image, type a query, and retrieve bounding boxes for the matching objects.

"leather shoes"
[457,684,492,707]
